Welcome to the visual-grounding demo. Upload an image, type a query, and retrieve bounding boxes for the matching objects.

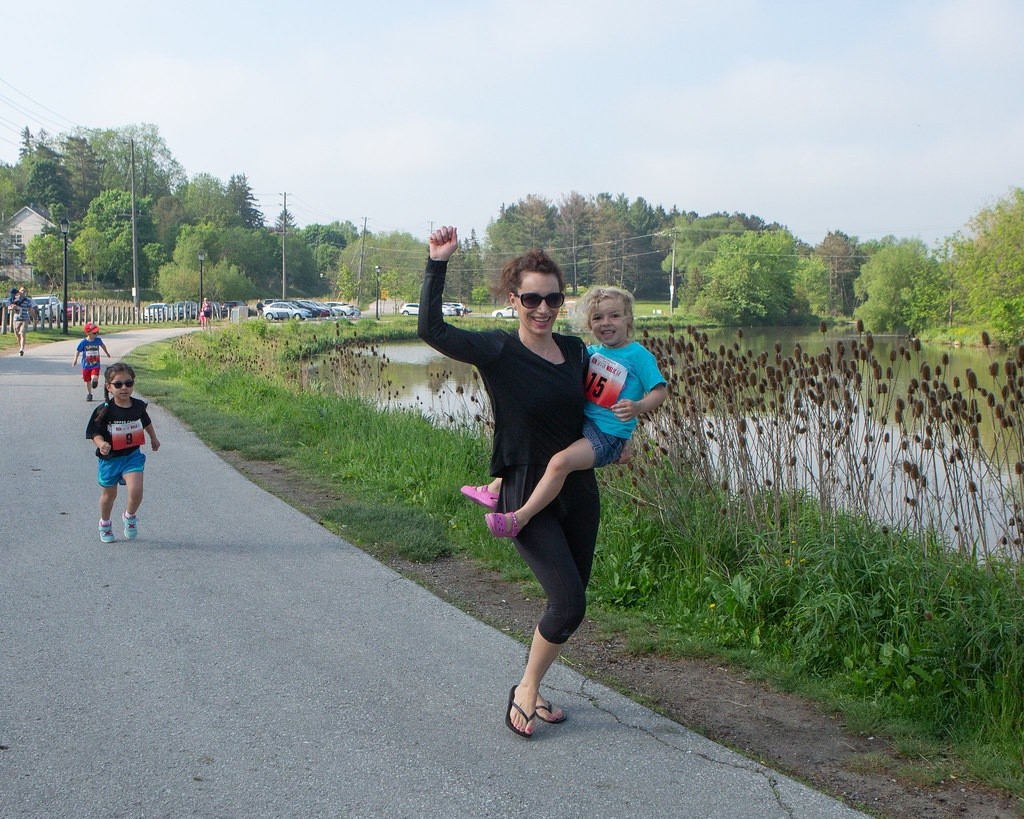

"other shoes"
[92,381,98,389]
[15,316,23,321]
[87,394,92,401]
[19,351,24,356]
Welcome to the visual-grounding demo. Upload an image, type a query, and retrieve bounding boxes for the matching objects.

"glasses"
[91,331,99,335]
[108,380,134,389]
[514,292,565,309]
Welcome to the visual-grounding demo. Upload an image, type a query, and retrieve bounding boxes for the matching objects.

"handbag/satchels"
[203,309,212,317]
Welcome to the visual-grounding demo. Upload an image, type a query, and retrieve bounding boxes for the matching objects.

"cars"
[442,302,473,316]
[66,302,85,322]
[141,302,172,321]
[220,300,251,318]
[31,296,64,322]
[171,301,198,319]
[399,303,420,316]
[262,298,361,320]
[262,301,313,321]
[202,301,222,317]
[491,306,519,319]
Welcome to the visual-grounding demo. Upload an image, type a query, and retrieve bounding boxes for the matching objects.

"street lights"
[196,249,206,312]
[60,217,70,335]
[374,266,381,320]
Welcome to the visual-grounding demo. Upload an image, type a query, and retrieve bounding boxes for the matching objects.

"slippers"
[504,684,536,739]
[536,701,568,725]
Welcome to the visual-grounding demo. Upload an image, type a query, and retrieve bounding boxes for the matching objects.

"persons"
[200,298,212,330]
[461,302,469,318]
[460,287,668,537]
[85,362,160,543]
[256,298,264,320]
[10,286,37,357]
[73,321,111,401]
[419,225,631,738]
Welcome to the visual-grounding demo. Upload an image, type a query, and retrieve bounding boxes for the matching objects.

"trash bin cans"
[231,307,248,324]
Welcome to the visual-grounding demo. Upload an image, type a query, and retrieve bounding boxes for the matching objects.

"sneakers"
[122,509,138,540]
[98,520,115,543]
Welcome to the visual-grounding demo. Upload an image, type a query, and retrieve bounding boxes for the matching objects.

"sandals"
[485,512,521,538]
[460,485,500,510]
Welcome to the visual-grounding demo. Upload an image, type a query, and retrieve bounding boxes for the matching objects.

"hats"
[84,323,99,333]
[11,288,19,294]
